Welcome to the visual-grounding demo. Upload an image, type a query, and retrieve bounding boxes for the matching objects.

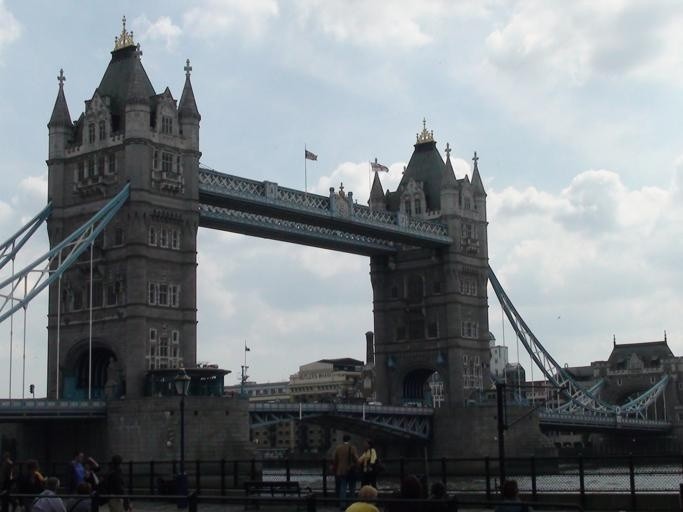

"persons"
[0,450,135,512]
[332,434,450,512]
[491,480,534,512]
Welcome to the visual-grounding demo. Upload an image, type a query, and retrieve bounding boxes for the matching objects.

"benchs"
[244,480,315,511]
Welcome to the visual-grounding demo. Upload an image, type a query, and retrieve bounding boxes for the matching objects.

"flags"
[371,161,389,173]
[245,345,250,351]
[305,150,317,160]
